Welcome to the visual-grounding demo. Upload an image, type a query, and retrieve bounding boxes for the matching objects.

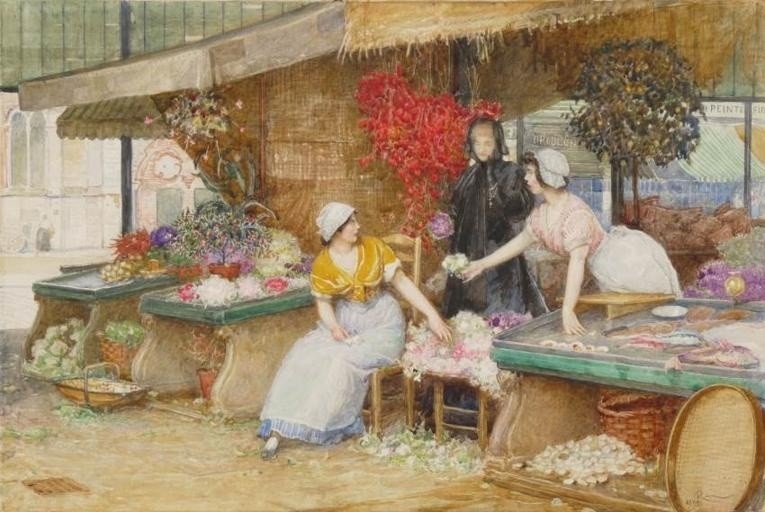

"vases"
[196,370,215,399]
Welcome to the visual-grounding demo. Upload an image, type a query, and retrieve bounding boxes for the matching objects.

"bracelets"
[488,182,498,205]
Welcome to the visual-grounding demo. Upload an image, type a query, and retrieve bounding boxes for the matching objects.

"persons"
[421,113,551,412]
[256,202,452,460]
[440,147,680,336]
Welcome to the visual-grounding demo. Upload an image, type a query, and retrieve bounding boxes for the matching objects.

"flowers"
[428,210,458,239]
[173,327,226,369]
[685,222,765,308]
[398,305,536,400]
[99,209,310,305]
[438,250,468,274]
[139,88,252,167]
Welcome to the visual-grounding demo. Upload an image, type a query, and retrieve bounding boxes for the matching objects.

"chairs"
[363,235,424,435]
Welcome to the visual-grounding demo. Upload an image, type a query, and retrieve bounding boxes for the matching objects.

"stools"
[425,366,497,455]
